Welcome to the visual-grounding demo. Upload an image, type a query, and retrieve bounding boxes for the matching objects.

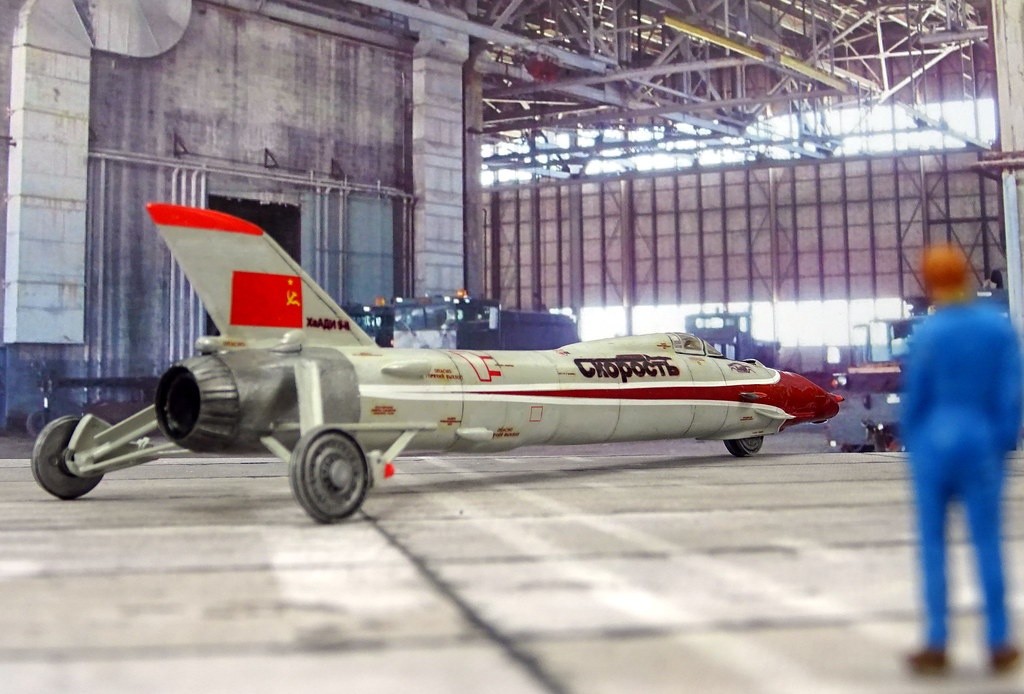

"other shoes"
[992,646,1018,673]
[907,650,947,672]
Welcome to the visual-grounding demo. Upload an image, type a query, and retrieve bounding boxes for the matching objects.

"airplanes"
[33,202,845,524]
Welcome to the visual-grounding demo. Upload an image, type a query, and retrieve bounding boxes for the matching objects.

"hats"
[919,246,971,303]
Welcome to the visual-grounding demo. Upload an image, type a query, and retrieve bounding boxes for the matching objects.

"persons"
[896,244,1024,676]
